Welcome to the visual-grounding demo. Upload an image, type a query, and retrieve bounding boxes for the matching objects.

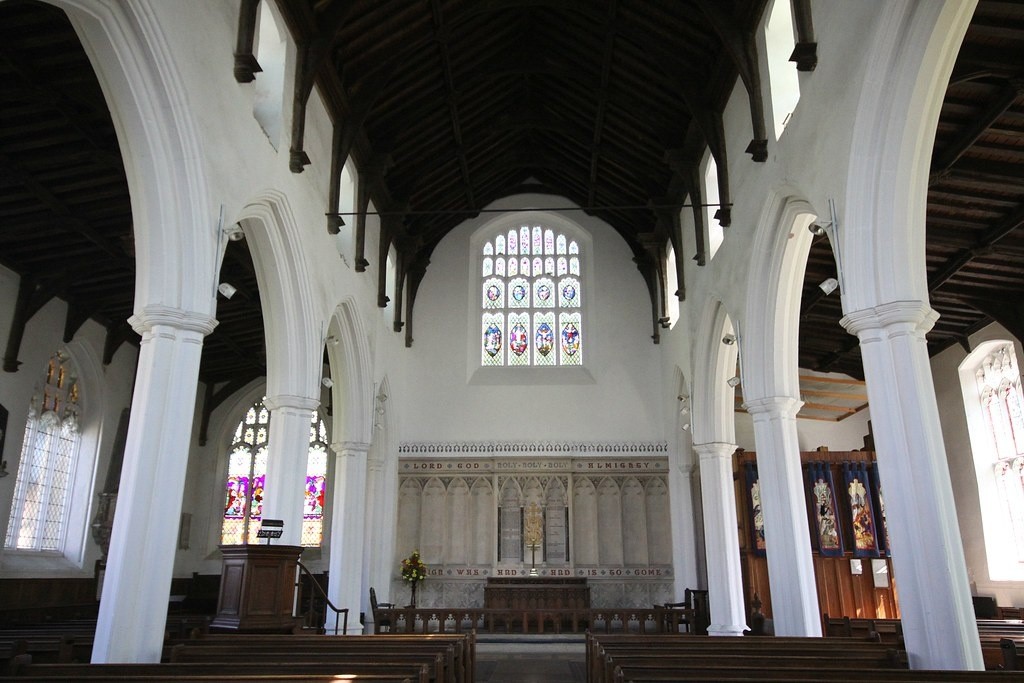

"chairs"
[369,586,398,632]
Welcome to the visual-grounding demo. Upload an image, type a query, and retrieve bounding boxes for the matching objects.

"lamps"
[677,381,695,436]
[808,198,845,299]
[371,382,389,436]
[211,202,246,299]
[317,318,339,390]
[721,317,744,391]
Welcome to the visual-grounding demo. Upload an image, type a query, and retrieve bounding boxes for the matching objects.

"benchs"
[664,588,711,634]
[585,619,1024,683]
[1,613,478,683]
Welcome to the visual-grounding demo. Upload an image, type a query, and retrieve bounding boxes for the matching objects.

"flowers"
[400,548,426,606]
